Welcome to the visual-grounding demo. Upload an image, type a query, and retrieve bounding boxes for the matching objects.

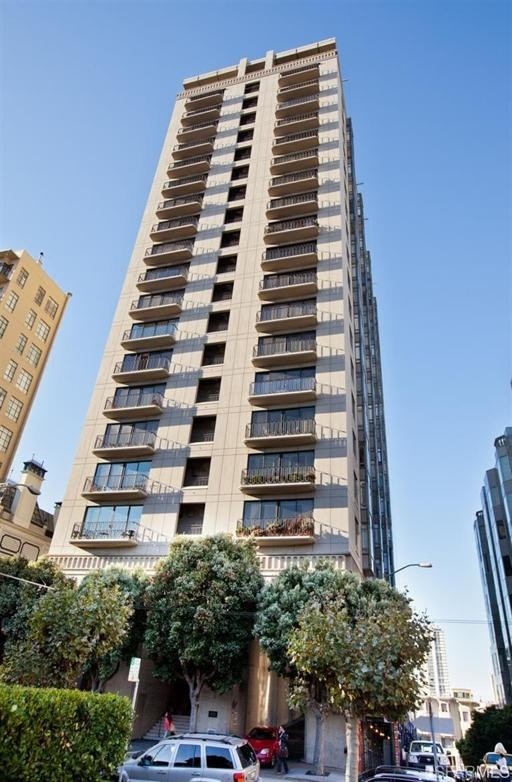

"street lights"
[383,563,432,582]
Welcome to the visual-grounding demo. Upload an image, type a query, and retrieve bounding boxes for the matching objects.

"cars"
[359,740,512,782]
[100,726,281,782]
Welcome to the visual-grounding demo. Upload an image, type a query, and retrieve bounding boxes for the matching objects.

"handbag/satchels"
[169,723,176,735]
[281,749,289,758]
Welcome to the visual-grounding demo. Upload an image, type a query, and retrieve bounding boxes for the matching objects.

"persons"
[495,752,510,782]
[162,712,170,737]
[275,724,290,775]
[167,715,174,733]
